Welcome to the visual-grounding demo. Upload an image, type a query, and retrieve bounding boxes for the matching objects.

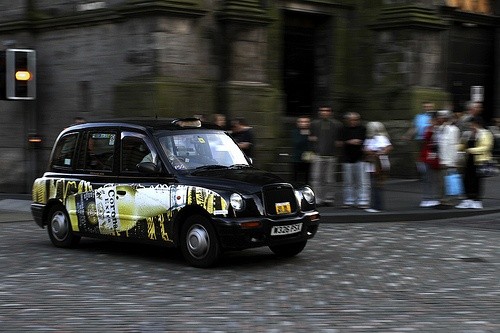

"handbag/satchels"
[445,169,462,195]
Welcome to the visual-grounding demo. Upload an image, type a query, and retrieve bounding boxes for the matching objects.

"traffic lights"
[5,47,37,101]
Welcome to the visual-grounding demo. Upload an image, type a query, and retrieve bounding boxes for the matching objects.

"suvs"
[28,116,321,269]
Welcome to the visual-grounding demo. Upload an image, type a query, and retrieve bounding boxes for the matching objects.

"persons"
[140,138,170,163]
[193,114,257,167]
[289,105,394,212]
[398,102,500,209]
[61,117,96,159]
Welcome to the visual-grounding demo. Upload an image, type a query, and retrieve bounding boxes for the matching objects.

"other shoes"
[366,209,381,213]
[358,206,371,208]
[323,202,333,207]
[455,200,471,208]
[470,201,483,208]
[420,200,439,207]
[340,205,351,208]
